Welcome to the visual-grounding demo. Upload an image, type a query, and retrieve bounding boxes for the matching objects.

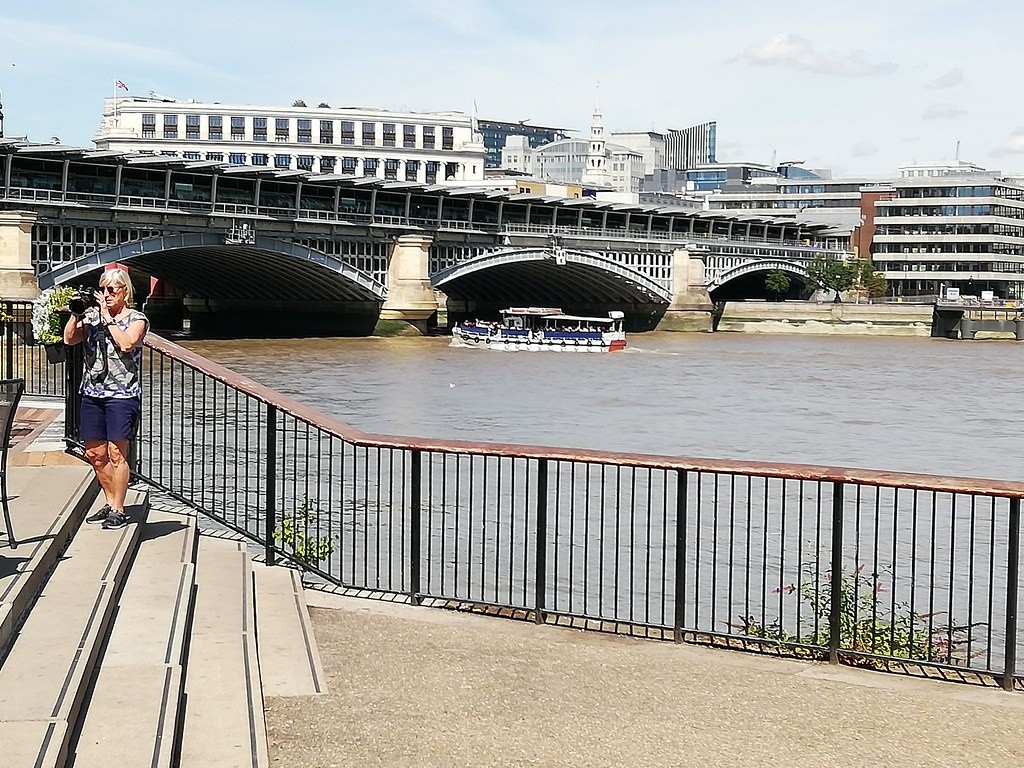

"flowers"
[0,296,13,322]
[31,284,77,345]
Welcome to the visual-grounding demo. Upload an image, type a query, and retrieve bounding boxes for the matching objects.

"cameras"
[69,287,106,316]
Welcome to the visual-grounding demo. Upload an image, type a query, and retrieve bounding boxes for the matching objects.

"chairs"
[0,377,25,549]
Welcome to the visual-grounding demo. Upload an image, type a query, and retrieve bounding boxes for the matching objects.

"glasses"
[98,284,126,293]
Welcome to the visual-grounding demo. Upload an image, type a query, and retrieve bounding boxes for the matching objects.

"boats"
[452,307,627,353]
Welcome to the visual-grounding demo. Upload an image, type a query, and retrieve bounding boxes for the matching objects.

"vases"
[0,321,5,336]
[45,342,66,364]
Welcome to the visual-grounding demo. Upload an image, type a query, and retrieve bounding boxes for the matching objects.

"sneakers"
[86,503,130,530]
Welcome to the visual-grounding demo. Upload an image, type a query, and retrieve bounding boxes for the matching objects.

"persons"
[63,269,151,531]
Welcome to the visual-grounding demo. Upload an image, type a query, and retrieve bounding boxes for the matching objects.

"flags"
[115,80,128,91]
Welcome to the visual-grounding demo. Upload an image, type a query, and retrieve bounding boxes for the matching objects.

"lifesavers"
[463,334,606,347]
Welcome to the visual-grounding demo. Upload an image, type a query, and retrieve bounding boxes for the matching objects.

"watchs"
[104,318,117,326]
